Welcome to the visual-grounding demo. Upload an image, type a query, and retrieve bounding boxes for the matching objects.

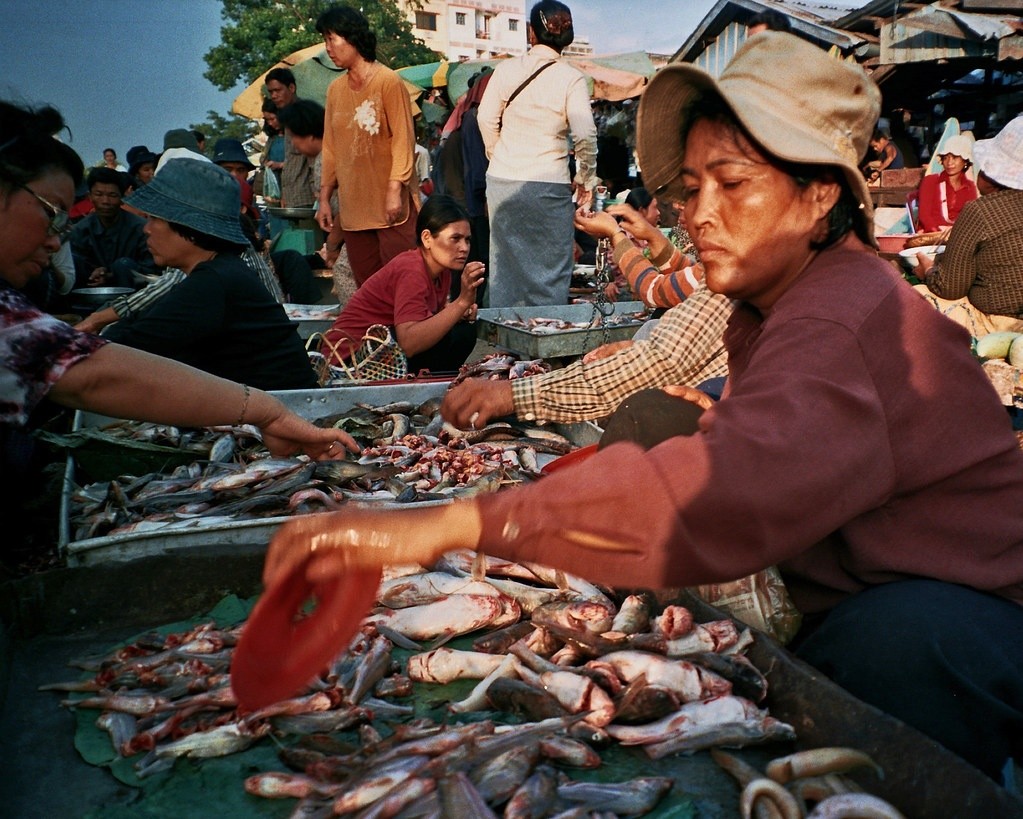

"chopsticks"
[927,228,951,252]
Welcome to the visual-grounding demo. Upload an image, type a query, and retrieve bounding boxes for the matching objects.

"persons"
[259,67,314,206]
[321,194,485,375]
[313,6,422,286]
[43,128,271,296]
[916,135,977,234]
[0,99,361,466]
[438,273,739,429]
[275,100,358,311]
[263,30,1023,784]
[869,128,903,179]
[476,0,598,307]
[746,9,790,38]
[911,116,1023,339]
[73,148,321,391]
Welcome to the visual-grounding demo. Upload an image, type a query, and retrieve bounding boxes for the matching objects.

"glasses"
[20,183,71,234]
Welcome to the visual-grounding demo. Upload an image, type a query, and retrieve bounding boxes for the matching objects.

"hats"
[211,137,257,172]
[636,30,883,251]
[122,148,251,244]
[162,129,204,154]
[127,145,157,173]
[974,114,1023,190]
[935,136,974,173]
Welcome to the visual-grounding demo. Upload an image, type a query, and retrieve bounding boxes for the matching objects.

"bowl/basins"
[874,234,912,250]
[73,288,135,307]
[898,245,946,266]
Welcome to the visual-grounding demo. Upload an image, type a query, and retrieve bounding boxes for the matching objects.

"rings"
[327,441,336,450]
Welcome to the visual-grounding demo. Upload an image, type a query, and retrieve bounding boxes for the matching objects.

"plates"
[232,544,383,711]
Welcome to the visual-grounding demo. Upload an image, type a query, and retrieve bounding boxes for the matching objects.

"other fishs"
[285,304,341,321]
[503,309,656,334]
[447,353,551,391]
[62,396,583,540]
[39,548,906,819]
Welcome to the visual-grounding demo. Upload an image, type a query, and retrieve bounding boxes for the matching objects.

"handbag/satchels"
[262,164,281,200]
[305,324,407,387]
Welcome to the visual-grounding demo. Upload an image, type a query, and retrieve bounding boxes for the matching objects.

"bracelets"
[232,384,250,427]
[609,228,626,243]
